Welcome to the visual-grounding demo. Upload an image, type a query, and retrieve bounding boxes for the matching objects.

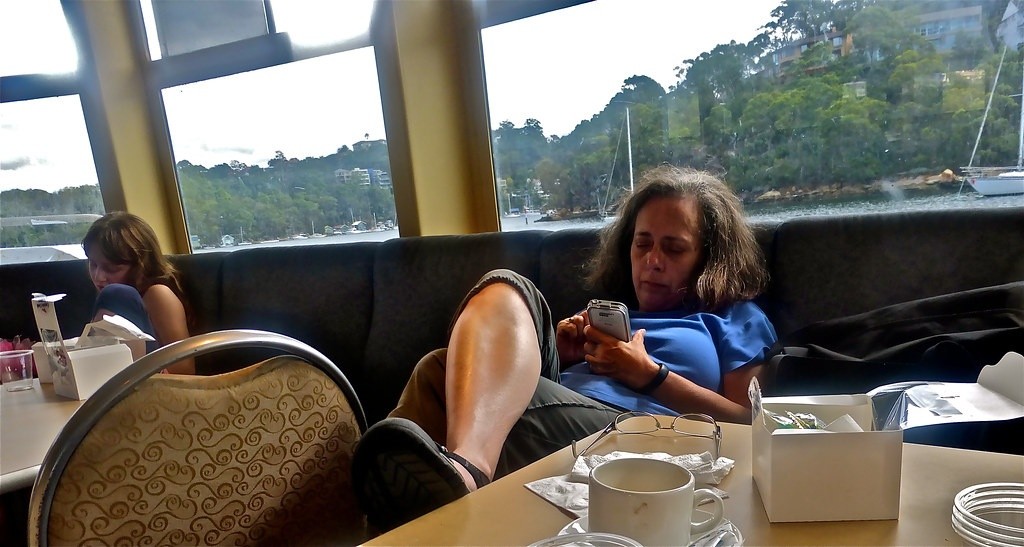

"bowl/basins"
[951,483,1024,547]
[527,532,643,546]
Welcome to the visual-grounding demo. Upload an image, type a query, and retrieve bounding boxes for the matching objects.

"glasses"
[570,409,721,464]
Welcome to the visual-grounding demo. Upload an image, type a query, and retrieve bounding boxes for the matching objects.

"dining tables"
[0,342,191,491]
[341,411,1024,547]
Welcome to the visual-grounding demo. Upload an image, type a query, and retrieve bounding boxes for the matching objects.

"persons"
[348,161,780,542]
[81,210,205,376]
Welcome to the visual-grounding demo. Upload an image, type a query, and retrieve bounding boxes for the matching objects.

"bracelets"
[646,363,669,390]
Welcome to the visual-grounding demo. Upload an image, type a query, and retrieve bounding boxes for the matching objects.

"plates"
[557,509,743,547]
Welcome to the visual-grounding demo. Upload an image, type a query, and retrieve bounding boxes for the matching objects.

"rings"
[568,315,575,324]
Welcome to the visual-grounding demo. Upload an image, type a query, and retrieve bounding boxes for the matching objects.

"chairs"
[24,325,377,547]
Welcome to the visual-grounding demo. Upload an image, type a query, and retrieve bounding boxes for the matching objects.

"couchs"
[0,196,1023,429]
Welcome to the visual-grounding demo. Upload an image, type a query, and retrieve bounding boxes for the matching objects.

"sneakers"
[348,417,492,528]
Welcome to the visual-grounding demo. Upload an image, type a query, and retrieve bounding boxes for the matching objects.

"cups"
[587,457,724,547]
[0,349,34,391]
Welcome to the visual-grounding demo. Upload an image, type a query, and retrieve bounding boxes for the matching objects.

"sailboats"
[962,0,1024,197]
[601,105,633,223]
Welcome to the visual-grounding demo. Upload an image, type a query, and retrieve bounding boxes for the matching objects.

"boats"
[186,208,402,251]
[500,191,558,218]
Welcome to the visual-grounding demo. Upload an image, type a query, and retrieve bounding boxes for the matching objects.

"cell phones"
[587,299,632,343]
